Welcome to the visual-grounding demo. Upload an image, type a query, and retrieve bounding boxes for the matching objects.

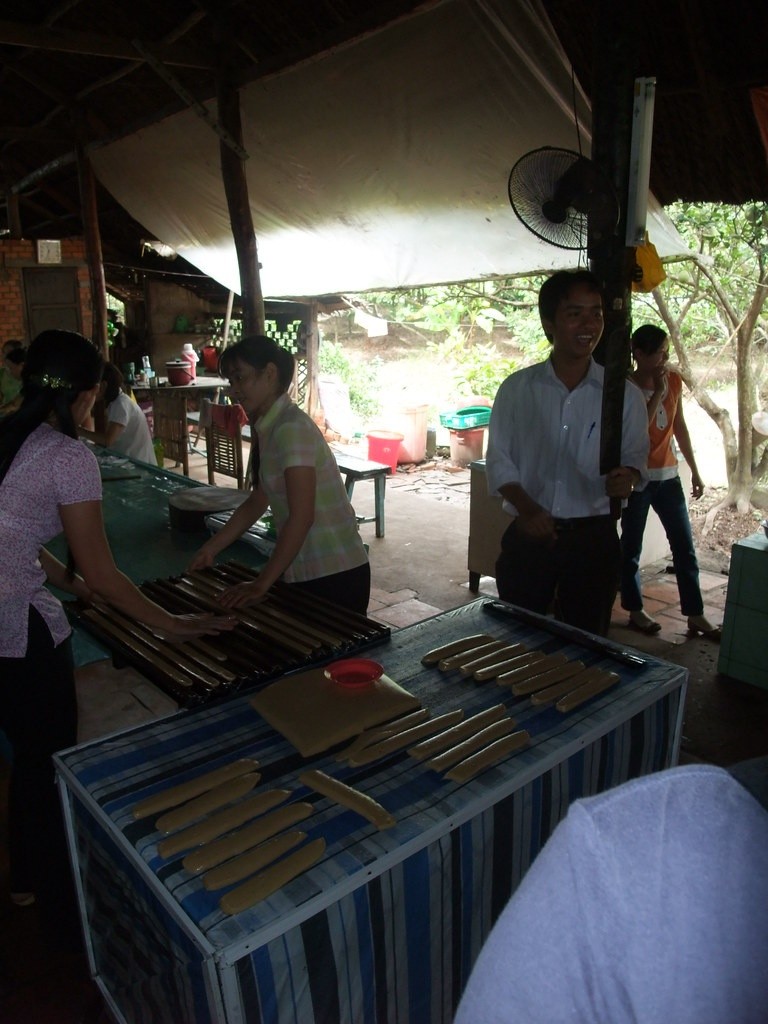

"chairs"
[203,397,250,489]
[149,384,207,479]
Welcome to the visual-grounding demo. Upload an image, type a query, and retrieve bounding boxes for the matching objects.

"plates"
[323,659,385,688]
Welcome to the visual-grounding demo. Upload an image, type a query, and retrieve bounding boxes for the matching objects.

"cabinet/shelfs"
[717,529,768,686]
[52,594,688,1023]
[468,460,517,594]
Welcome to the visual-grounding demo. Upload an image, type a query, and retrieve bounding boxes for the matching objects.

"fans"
[507,144,622,258]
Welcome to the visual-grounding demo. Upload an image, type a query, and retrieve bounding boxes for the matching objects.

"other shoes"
[687,617,722,644]
[627,610,662,635]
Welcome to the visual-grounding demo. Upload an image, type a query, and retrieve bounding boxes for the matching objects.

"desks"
[132,376,231,454]
[45,442,280,665]
[63,560,390,709]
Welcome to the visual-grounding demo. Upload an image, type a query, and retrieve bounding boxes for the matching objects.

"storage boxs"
[440,407,493,430]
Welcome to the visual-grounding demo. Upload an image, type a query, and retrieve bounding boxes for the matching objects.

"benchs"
[185,412,392,538]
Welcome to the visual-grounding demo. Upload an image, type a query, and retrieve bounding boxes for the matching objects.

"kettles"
[181,343,200,379]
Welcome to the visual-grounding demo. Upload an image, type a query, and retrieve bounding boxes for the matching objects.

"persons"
[188,335,371,615]
[1,308,240,907]
[495,269,722,640]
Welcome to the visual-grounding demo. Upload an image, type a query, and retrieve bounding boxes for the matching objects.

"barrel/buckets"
[164,361,193,386]
[450,429,483,467]
[389,401,432,464]
[365,428,404,473]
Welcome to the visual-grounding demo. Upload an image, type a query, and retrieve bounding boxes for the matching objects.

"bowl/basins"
[455,406,492,415]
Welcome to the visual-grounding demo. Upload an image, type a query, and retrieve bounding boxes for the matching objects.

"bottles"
[175,314,202,334]
[153,435,165,468]
[126,356,157,387]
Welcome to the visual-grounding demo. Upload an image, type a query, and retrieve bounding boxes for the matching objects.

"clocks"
[37,239,62,264]
